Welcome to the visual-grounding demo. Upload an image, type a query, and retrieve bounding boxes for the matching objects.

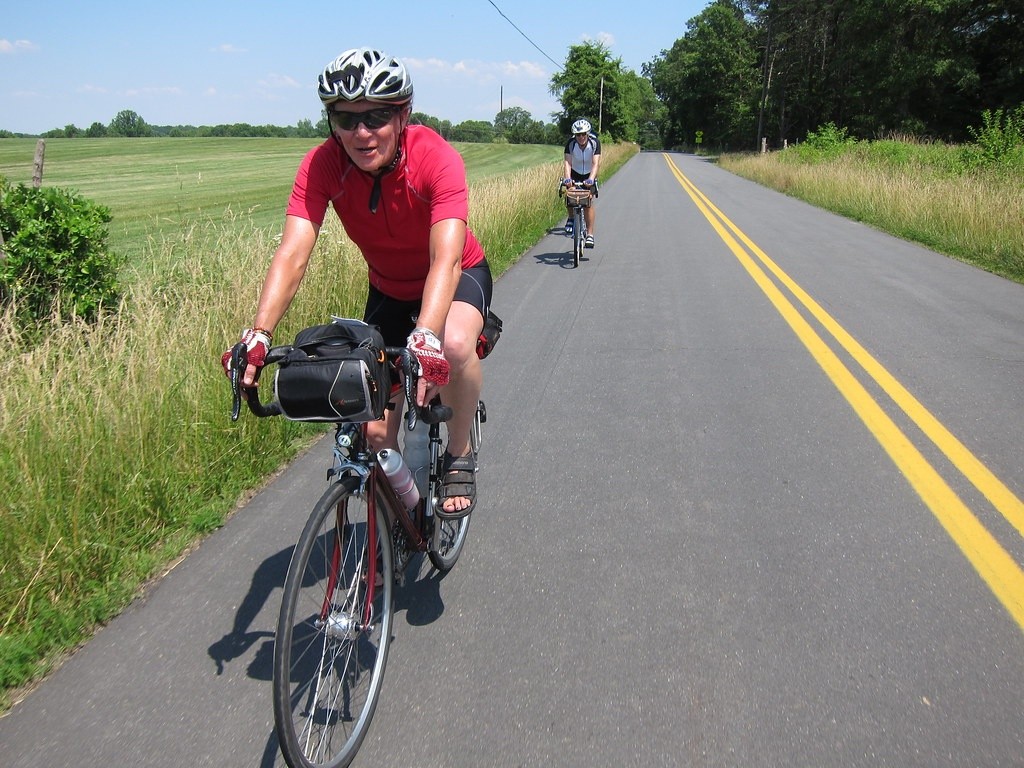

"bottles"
[376,447,419,510]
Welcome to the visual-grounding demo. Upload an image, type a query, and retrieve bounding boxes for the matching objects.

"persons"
[220,47,494,592]
[564,119,601,247]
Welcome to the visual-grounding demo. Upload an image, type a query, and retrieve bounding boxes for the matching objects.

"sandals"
[359,542,383,590]
[564,218,574,233]
[435,442,477,520]
[585,236,594,247]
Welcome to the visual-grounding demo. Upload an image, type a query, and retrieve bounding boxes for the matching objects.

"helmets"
[571,119,591,134]
[317,48,414,105]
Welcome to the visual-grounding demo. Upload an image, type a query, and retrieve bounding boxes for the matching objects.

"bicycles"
[558,179,599,267]
[231,311,485,768]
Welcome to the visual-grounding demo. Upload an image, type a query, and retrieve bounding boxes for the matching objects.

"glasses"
[327,105,401,130]
[577,132,586,136]
